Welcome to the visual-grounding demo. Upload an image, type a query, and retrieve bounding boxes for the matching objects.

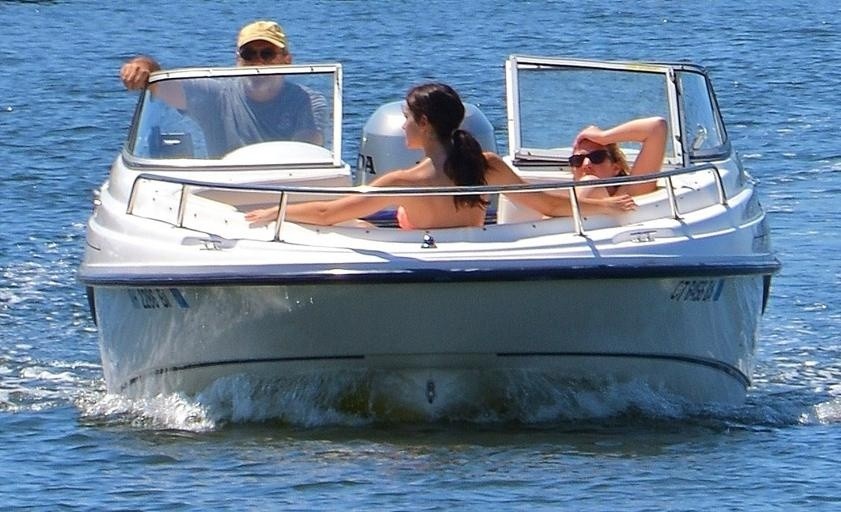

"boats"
[70,49,783,434]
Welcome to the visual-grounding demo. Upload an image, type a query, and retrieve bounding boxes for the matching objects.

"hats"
[238,21,287,48]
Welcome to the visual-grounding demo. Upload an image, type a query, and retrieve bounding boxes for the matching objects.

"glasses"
[568,150,611,167]
[239,47,283,62]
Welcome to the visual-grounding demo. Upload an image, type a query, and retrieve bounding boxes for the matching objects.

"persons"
[120,20,329,160]
[244,82,640,229]
[482,117,667,218]
